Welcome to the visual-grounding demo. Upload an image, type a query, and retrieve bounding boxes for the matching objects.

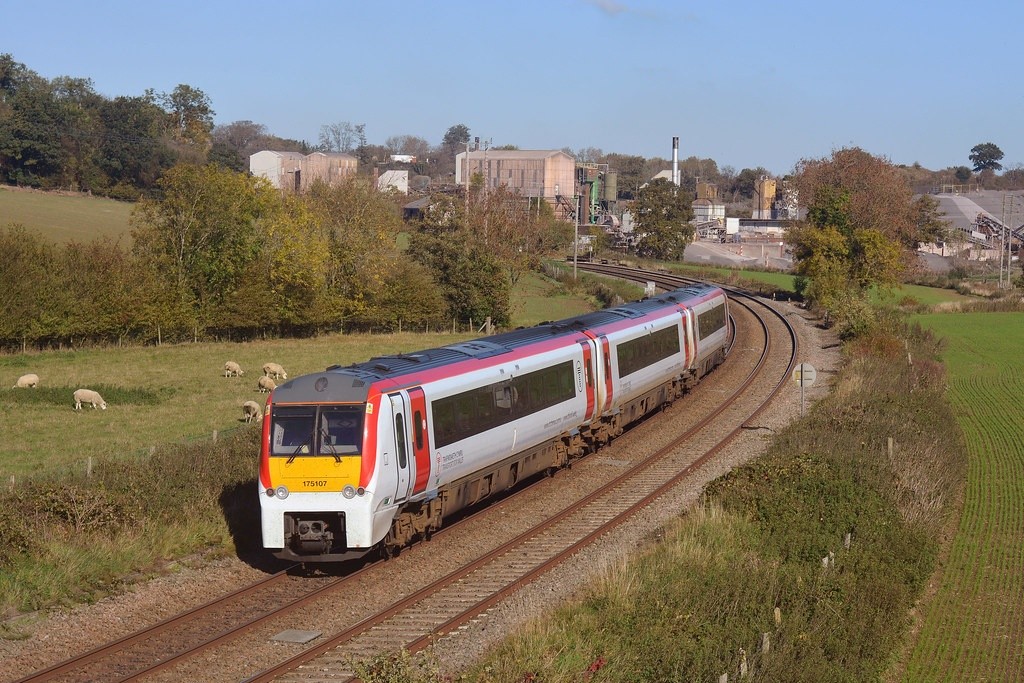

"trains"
[258,283,730,563]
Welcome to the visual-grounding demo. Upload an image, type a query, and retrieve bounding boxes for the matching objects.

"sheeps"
[243,400,263,424]
[73,389,106,411]
[11,373,40,389]
[221,361,244,378]
[258,375,278,393]
[263,362,287,380]
